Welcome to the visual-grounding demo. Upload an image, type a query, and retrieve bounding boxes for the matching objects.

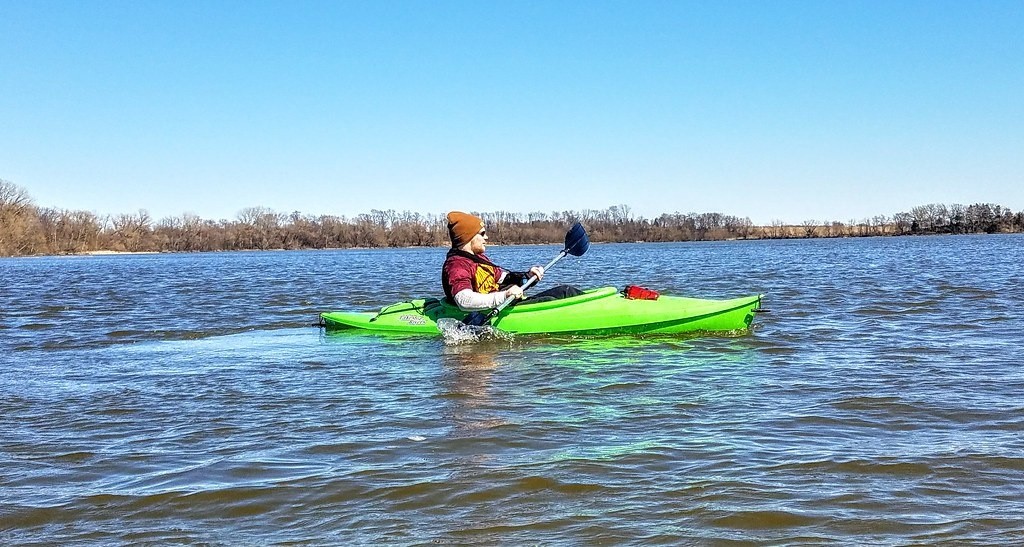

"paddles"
[455,220,590,342]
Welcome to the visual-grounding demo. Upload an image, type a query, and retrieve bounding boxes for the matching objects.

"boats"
[319,284,766,339]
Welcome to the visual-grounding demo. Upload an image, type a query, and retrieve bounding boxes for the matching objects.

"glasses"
[477,231,486,237]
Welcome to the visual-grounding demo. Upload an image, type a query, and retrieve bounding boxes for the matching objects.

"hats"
[447,212,485,249]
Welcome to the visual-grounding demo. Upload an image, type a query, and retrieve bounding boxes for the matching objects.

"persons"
[442,211,585,310]
